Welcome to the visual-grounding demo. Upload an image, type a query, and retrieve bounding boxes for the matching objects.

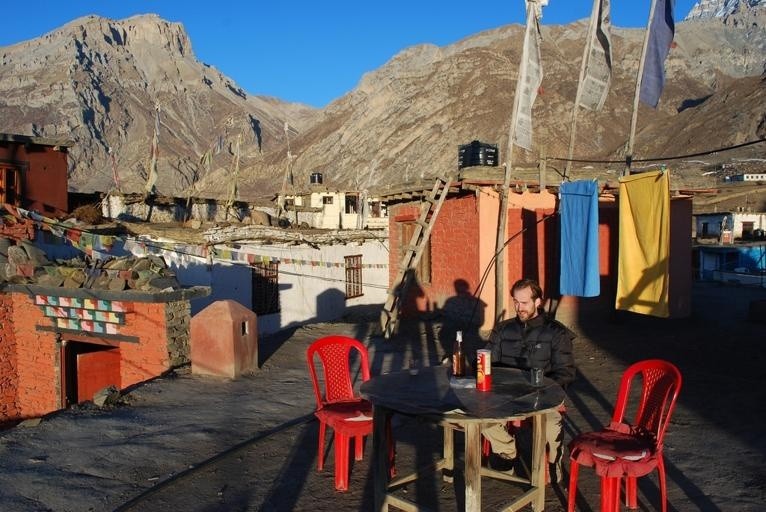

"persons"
[478,279,578,485]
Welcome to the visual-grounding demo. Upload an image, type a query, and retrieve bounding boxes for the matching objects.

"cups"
[529,366,546,388]
[530,391,543,409]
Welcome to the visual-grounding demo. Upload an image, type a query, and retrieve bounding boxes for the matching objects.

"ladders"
[381,172,453,340]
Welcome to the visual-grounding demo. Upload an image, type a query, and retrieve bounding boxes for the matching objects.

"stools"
[481,402,567,489]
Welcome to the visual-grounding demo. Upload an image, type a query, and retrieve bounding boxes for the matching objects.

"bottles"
[450,328,468,378]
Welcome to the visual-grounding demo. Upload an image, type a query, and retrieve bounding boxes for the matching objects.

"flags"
[142,110,161,192]
[191,146,215,194]
[637,2,676,112]
[573,1,616,118]
[109,156,120,194]
[510,0,551,154]
[277,151,295,214]
[226,139,241,205]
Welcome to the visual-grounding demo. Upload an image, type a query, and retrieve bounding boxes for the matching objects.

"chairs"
[305,336,396,491]
[565,360,682,510]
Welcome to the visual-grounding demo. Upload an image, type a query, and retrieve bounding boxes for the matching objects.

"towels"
[614,170,673,319]
[558,179,600,299]
